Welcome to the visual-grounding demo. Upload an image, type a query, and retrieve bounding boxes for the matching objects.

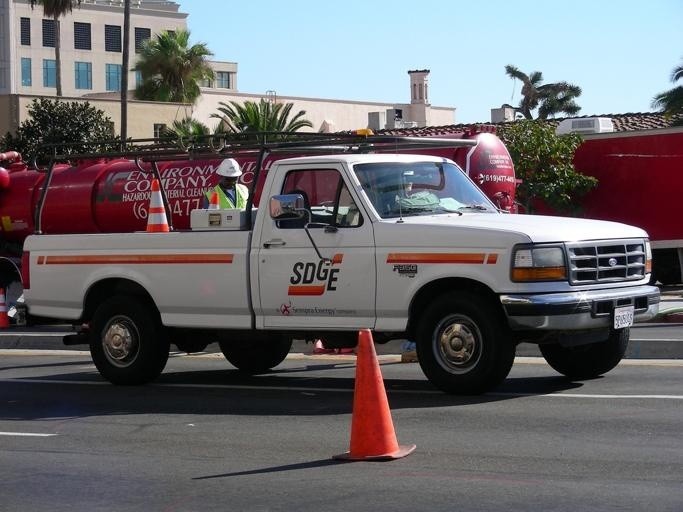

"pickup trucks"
[18,131,662,403]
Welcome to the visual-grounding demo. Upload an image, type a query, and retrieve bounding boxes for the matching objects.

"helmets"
[215,158,244,178]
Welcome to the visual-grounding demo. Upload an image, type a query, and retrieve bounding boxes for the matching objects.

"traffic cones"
[0,286,11,330]
[330,320,420,465]
[142,176,173,238]
[206,192,224,209]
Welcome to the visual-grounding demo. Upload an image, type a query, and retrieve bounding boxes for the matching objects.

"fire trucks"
[363,114,682,285]
[0,113,519,324]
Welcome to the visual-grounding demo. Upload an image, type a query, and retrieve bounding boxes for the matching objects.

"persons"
[384,168,413,216]
[204,158,255,212]
